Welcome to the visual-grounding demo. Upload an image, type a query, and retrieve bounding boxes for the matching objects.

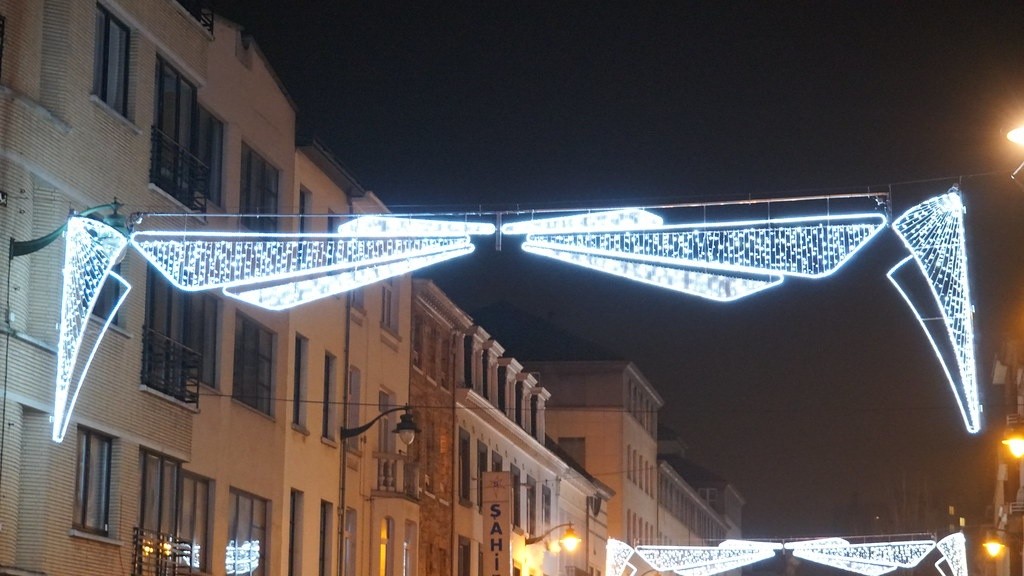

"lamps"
[10,197,133,266]
[982,525,1024,557]
[525,522,582,552]
[1001,423,1024,458]
[340,403,422,446]
